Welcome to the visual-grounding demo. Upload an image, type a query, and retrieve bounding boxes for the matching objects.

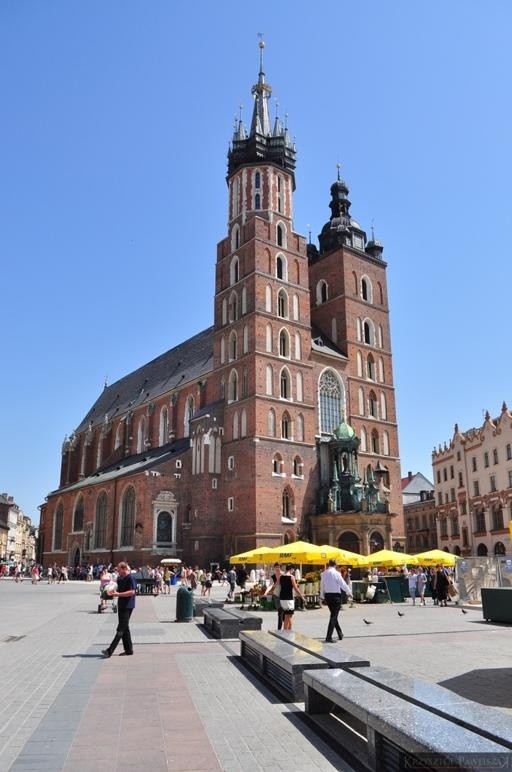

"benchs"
[194,597,262,640]
[302,666,511,770]
[239,630,370,703]
[481,588,511,621]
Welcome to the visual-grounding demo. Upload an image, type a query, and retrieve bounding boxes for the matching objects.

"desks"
[244,573,431,610]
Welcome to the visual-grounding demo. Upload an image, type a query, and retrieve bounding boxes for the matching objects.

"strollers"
[98,583,117,613]
[240,579,267,610]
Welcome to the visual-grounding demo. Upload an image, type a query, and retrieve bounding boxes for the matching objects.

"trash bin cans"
[175,586,195,623]
[479,586,512,627]
[368,576,405,603]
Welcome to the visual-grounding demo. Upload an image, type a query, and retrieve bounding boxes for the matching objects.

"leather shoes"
[119,651,133,656]
[339,634,343,639]
[326,638,336,643]
[102,650,110,658]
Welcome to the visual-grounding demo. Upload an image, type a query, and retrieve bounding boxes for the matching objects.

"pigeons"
[363,619,374,625]
[397,611,405,617]
[461,608,469,614]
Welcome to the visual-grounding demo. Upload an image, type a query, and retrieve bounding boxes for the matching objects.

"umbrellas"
[252,540,338,578]
[229,546,274,570]
[318,544,369,572]
[356,549,420,574]
[412,548,464,568]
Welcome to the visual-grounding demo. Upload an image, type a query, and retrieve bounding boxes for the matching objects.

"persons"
[277,562,306,630]
[361,566,408,583]
[407,562,456,607]
[99,566,113,611]
[100,560,138,657]
[1,558,249,594]
[342,453,348,473]
[318,558,354,644]
[340,566,351,588]
[262,561,286,630]
